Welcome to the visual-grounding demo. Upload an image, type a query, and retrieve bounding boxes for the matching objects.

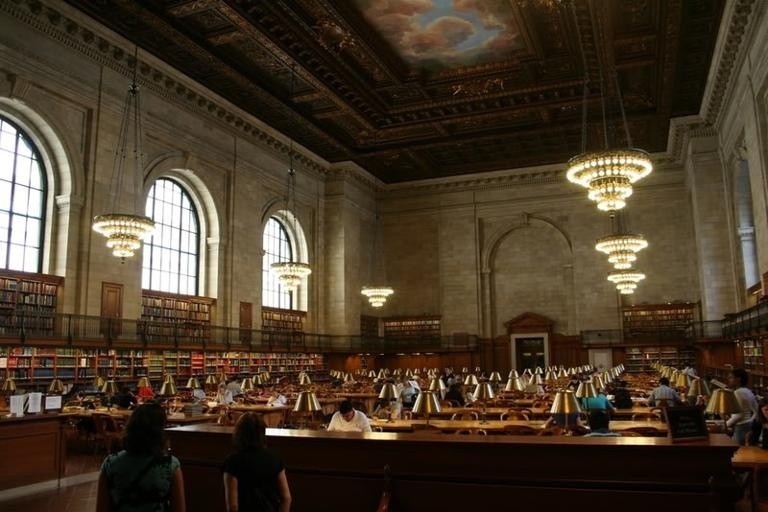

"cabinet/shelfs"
[620,302,697,372]
[0,270,64,336]
[262,306,307,347]
[742,340,765,372]
[140,289,216,344]
[361,314,441,347]
[0,345,327,400]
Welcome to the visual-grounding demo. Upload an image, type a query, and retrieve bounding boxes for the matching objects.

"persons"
[193,384,206,398]
[380,375,420,407]
[217,376,242,405]
[541,410,621,437]
[113,385,137,409]
[582,381,632,410]
[222,412,292,512]
[97,403,186,512]
[647,377,679,406]
[268,390,287,406]
[139,383,155,398]
[726,368,768,450]
[327,400,372,432]
[439,374,465,407]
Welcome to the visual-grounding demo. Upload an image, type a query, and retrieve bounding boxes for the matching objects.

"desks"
[63,374,768,512]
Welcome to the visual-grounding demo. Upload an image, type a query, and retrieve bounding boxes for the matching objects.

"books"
[623,309,698,372]
[261,310,305,352]
[733,337,764,395]
[384,320,441,348]
[141,295,211,344]
[0,344,323,379]
[0,278,57,338]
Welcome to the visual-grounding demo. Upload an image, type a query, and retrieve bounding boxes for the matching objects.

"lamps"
[270,62,312,292]
[606,271,647,294]
[593,206,650,270]
[564,9,655,217]
[360,131,394,308]
[92,11,155,263]
